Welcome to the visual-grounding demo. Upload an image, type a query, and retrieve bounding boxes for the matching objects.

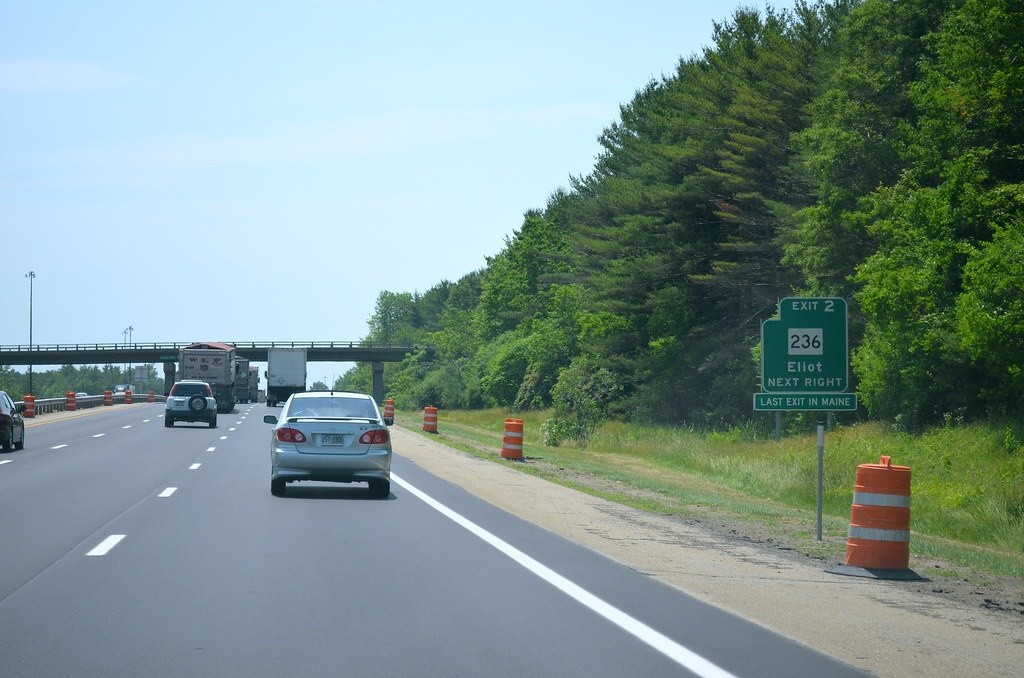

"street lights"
[121,328,128,384]
[128,326,135,384]
[25,270,36,395]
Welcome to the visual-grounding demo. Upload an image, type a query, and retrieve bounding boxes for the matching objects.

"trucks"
[114,384,135,394]
[265,347,308,408]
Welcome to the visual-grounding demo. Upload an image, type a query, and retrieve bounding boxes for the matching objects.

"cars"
[76,393,87,397]
[0,389,27,453]
[262,389,393,498]
[164,379,218,429]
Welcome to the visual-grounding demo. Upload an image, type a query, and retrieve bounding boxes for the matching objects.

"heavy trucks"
[235,355,251,403]
[179,341,240,414]
[248,367,261,404]
